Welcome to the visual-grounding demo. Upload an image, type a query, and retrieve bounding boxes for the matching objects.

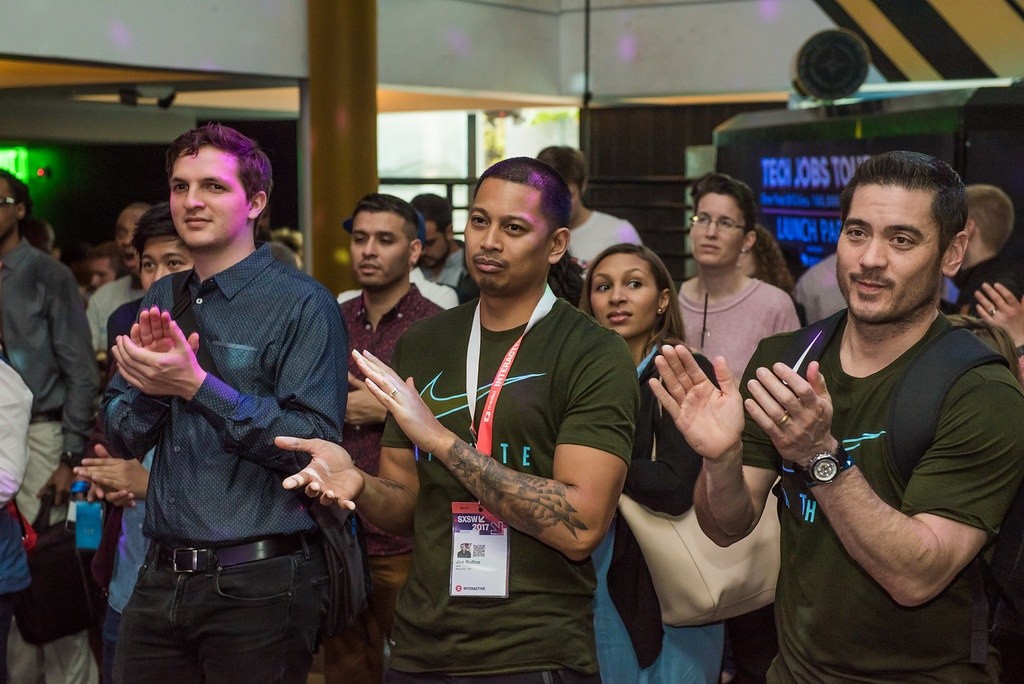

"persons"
[0,122,1024,684]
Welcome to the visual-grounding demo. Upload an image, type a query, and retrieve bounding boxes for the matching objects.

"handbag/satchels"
[616,377,781,626]
[14,486,104,643]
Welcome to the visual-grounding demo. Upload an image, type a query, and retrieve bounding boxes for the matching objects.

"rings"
[388,388,399,398]
[776,413,789,426]
[990,308,997,316]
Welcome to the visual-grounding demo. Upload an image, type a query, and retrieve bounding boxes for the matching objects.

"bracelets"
[1016,344,1024,357]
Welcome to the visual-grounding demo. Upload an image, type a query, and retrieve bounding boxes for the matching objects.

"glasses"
[0,198,17,206]
[689,215,744,230]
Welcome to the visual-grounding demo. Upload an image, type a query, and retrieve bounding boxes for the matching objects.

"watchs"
[791,446,848,483]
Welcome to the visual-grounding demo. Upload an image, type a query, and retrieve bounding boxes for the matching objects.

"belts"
[158,532,320,574]
[30,410,61,423]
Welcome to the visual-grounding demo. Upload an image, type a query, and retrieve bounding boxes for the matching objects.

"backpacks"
[788,308,1024,665]
